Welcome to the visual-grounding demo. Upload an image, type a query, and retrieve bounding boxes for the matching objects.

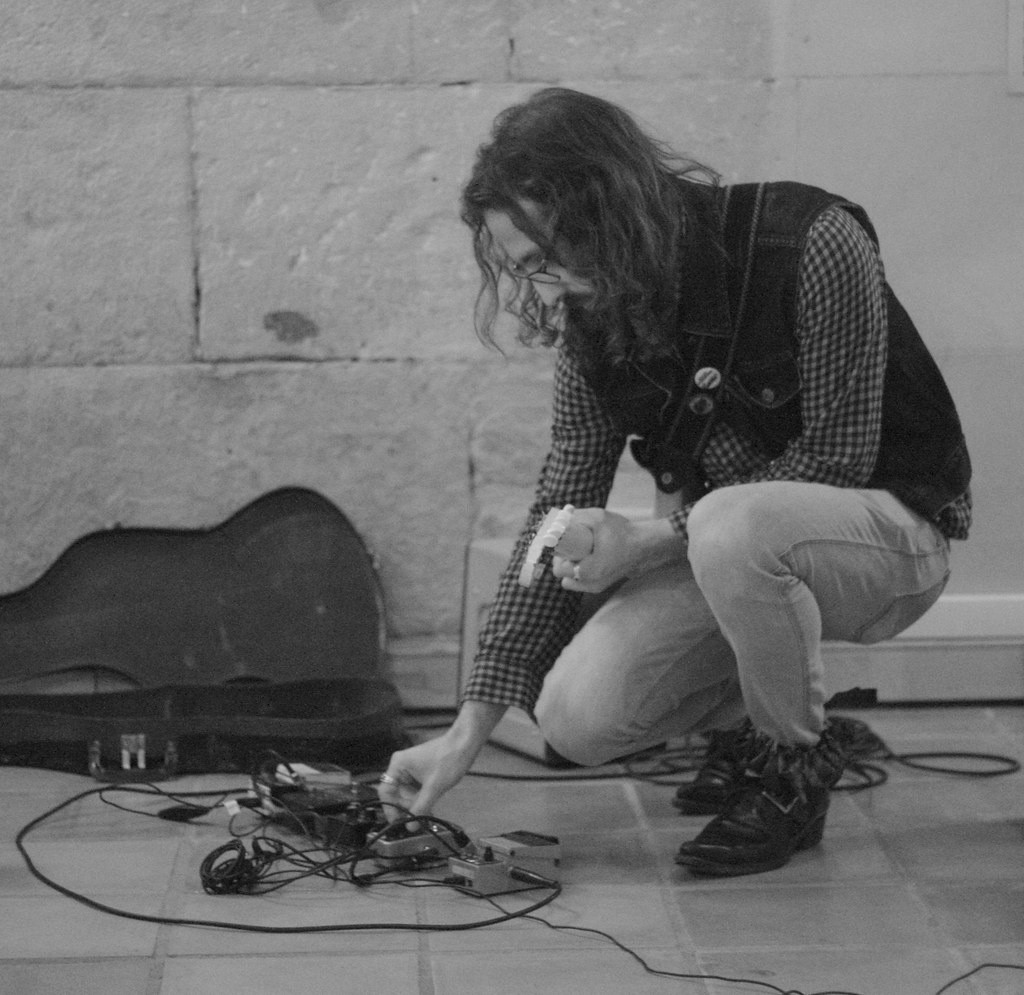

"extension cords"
[366,812,468,871]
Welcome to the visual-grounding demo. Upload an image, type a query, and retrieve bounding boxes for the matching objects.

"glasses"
[509,216,564,283]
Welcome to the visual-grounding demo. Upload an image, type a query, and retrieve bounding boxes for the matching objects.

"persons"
[378,87,972,877]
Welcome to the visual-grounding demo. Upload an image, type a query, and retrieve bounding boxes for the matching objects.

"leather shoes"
[674,734,844,876]
[672,718,755,815]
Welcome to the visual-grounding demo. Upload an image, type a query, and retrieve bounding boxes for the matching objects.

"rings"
[573,565,580,581]
[380,774,399,786]
